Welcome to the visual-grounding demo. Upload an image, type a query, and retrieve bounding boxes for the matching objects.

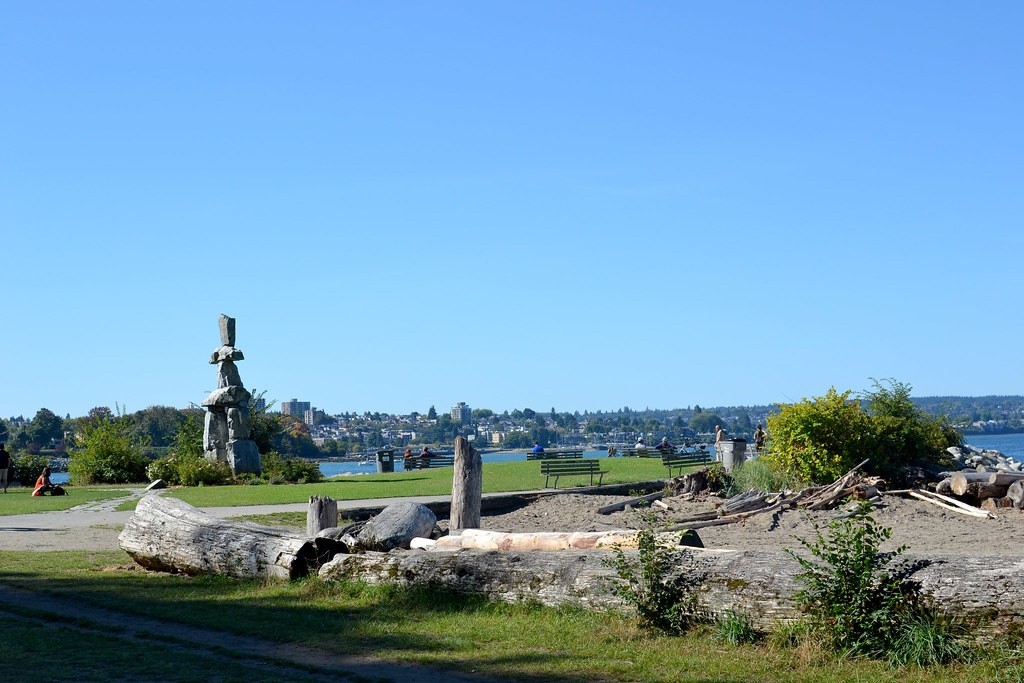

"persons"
[0,442,10,494]
[532,442,545,459]
[753,424,766,452]
[31,467,59,497]
[654,436,675,458]
[635,437,646,457]
[713,425,726,461]
[404,449,416,471]
[413,446,435,469]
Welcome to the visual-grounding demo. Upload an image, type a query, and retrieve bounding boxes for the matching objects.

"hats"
[637,437,643,442]
[661,437,668,441]
[423,447,429,450]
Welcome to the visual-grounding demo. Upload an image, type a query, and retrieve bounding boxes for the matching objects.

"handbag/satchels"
[50,486,65,495]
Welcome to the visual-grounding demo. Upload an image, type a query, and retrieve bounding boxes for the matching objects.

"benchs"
[527,450,583,461]
[404,456,455,471]
[621,448,672,458]
[661,451,721,478]
[540,459,610,489]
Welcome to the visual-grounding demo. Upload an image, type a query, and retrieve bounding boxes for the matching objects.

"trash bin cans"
[374,448,396,474]
[718,437,748,474]
